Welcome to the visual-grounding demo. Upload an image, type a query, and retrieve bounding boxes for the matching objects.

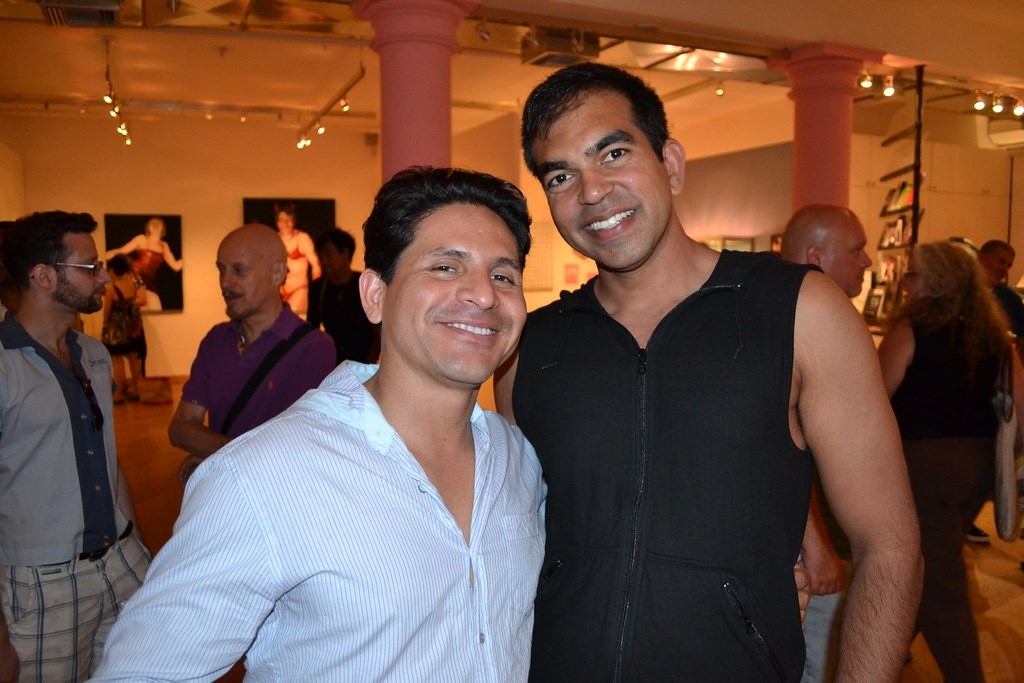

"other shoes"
[964,523,991,542]
[125,389,140,402]
[113,395,124,405]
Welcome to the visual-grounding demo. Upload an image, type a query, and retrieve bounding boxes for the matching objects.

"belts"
[36,520,134,567]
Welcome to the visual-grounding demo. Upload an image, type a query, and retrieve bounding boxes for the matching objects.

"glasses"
[30,261,104,279]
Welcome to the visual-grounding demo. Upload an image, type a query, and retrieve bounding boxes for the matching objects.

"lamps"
[973,91,985,110]
[104,86,131,146]
[881,75,894,96]
[992,93,1003,113]
[860,75,872,88]
[1012,99,1024,115]
[716,81,724,96]
[296,97,349,148]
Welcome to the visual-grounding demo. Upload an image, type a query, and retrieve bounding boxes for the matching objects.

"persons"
[105,217,182,312]
[306,226,381,367]
[1,210,155,682]
[167,225,339,461]
[97,254,148,406]
[83,165,816,683]
[876,237,1024,683]
[490,59,927,683]
[273,198,322,321]
[787,201,877,683]
